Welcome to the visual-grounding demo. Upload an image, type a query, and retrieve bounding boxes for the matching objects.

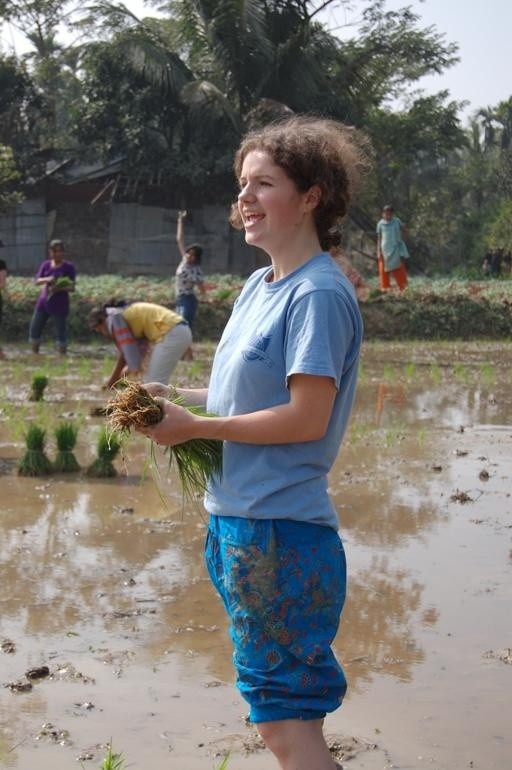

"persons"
[481,257,491,270]
[132,109,375,770]
[374,206,412,291]
[173,208,213,361]
[88,295,193,393]
[28,239,78,355]
[0,240,12,363]
[329,245,371,298]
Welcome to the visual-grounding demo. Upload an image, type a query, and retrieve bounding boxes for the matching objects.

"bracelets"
[167,384,180,399]
